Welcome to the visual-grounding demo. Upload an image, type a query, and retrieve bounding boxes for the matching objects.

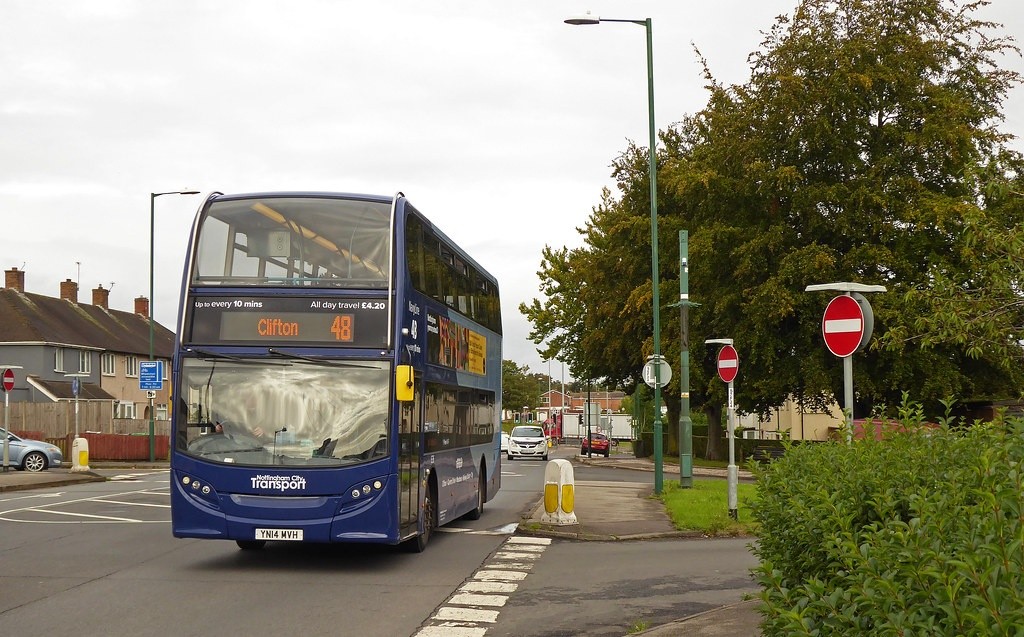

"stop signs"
[822,295,865,358]
[3,369,15,392]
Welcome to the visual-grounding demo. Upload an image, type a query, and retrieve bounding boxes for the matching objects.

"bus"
[167,189,504,552]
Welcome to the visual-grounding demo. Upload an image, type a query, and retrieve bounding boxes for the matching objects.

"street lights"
[716,345,739,383]
[146,191,201,464]
[561,15,667,497]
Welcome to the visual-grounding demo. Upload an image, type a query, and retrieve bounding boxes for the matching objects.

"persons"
[214,390,264,438]
[441,316,469,370]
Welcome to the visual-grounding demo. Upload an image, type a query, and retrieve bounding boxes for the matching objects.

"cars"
[580,432,609,458]
[500,430,510,452]
[0,427,64,472]
[505,424,551,461]
